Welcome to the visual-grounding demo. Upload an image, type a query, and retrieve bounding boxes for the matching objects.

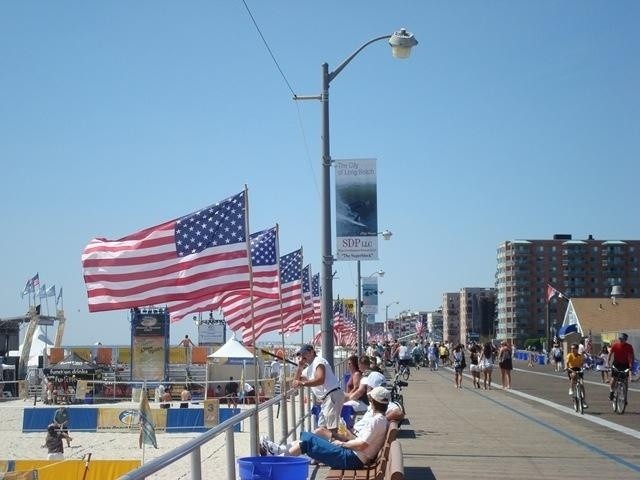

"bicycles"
[607,364,631,416]
[393,364,410,382]
[385,358,408,428]
[561,366,590,415]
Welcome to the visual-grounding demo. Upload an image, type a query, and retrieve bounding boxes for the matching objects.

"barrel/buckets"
[514,349,545,364]
[237,456,311,480]
[311,372,352,430]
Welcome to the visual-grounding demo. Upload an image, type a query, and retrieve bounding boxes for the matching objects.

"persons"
[292,345,404,464]
[578,339,594,370]
[159,379,173,402]
[366,339,453,375]
[527,346,537,367]
[553,345,562,372]
[46,380,71,403]
[271,357,280,380]
[215,377,255,408]
[181,386,191,401]
[565,344,587,407]
[176,334,198,364]
[454,341,516,390]
[46,419,72,459]
[597,333,635,405]
[262,386,392,470]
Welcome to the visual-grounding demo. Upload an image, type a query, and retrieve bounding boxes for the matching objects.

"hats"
[360,371,390,404]
[297,345,313,355]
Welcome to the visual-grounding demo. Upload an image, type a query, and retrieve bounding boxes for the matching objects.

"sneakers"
[261,436,283,455]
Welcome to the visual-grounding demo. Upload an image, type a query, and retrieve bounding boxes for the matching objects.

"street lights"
[363,268,386,346]
[358,228,392,357]
[385,300,401,334]
[320,28,421,371]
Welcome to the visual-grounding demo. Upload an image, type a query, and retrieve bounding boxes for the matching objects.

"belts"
[324,387,340,399]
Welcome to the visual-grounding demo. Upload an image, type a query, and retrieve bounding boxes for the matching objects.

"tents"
[43,352,103,403]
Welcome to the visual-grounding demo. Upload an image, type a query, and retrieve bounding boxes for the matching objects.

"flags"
[19,273,63,302]
[165,224,284,325]
[221,245,359,349]
[80,190,253,314]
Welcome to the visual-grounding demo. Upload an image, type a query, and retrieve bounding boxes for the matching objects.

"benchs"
[323,414,408,480]
